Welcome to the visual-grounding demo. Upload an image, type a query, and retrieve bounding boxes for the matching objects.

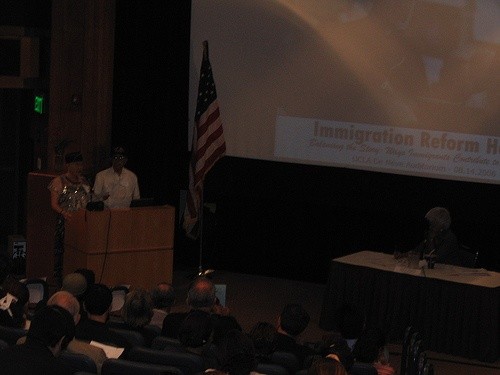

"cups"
[425,255,437,269]
[408,252,419,268]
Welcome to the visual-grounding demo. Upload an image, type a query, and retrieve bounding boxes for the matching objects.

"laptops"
[130,198,152,207]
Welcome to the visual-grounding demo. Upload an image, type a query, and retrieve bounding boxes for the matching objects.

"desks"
[318,250,500,364]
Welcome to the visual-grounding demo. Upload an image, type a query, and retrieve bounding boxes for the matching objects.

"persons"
[0,268,395,375]
[394,206,457,264]
[48,151,91,289]
[92,147,140,208]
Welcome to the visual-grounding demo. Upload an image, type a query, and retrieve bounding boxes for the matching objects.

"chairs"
[0,319,378,375]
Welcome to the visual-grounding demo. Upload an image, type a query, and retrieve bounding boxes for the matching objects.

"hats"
[61,273,88,297]
[426,207,452,219]
[65,152,83,163]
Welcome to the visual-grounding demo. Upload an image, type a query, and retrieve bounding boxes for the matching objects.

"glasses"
[113,155,125,160]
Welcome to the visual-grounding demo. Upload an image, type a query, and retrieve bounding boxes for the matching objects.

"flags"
[183,40,230,282]
